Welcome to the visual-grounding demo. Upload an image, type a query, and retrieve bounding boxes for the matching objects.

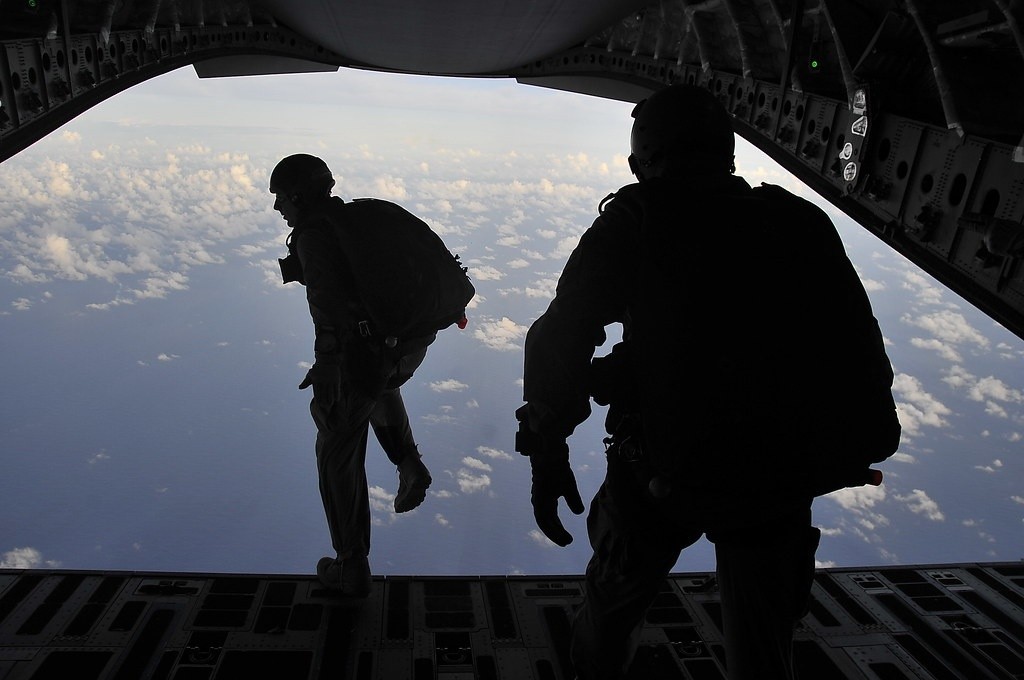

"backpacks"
[291,198,476,360]
[607,176,901,501]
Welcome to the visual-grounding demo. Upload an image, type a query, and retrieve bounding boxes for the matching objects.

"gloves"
[349,345,386,411]
[530,439,583,547]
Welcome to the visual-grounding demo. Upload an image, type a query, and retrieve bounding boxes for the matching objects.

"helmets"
[627,81,736,187]
[270,153,335,203]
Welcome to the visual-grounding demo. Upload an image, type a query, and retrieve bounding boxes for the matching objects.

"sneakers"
[317,548,369,601]
[396,453,430,513]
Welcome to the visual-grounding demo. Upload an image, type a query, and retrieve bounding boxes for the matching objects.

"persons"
[513,86,868,680]
[269,154,432,597]
